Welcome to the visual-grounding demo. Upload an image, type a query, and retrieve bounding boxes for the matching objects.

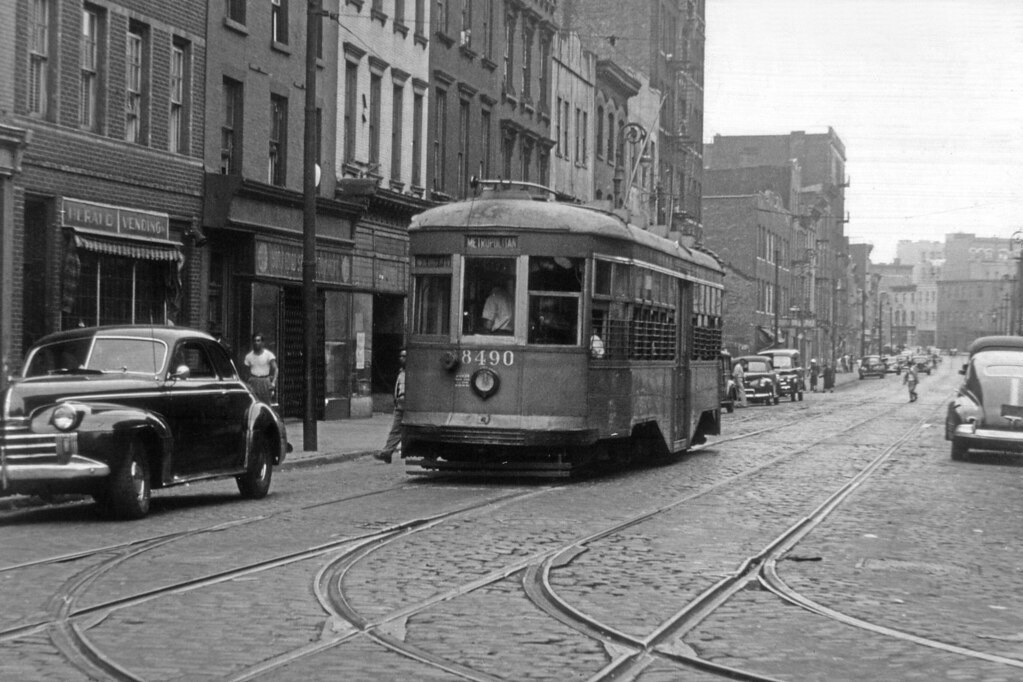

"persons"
[374,350,406,463]
[732,359,747,407]
[244,332,278,408]
[807,359,835,393]
[903,362,919,402]
[478,273,514,335]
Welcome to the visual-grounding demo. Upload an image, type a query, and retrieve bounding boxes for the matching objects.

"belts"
[250,374,270,378]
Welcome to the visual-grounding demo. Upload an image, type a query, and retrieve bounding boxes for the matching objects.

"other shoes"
[908,398,916,402]
[373,450,391,463]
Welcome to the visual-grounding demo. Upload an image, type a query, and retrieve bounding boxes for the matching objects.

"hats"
[554,256,572,269]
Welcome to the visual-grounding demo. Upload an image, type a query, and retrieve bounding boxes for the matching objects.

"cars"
[945,335,1023,460]
[721,349,781,412]
[758,349,806,402]
[886,347,943,376]
[858,355,887,380]
[0,324,293,520]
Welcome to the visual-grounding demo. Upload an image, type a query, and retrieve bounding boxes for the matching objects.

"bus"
[401,177,727,478]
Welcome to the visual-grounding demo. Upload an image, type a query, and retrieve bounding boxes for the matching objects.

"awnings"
[65,227,183,261]
